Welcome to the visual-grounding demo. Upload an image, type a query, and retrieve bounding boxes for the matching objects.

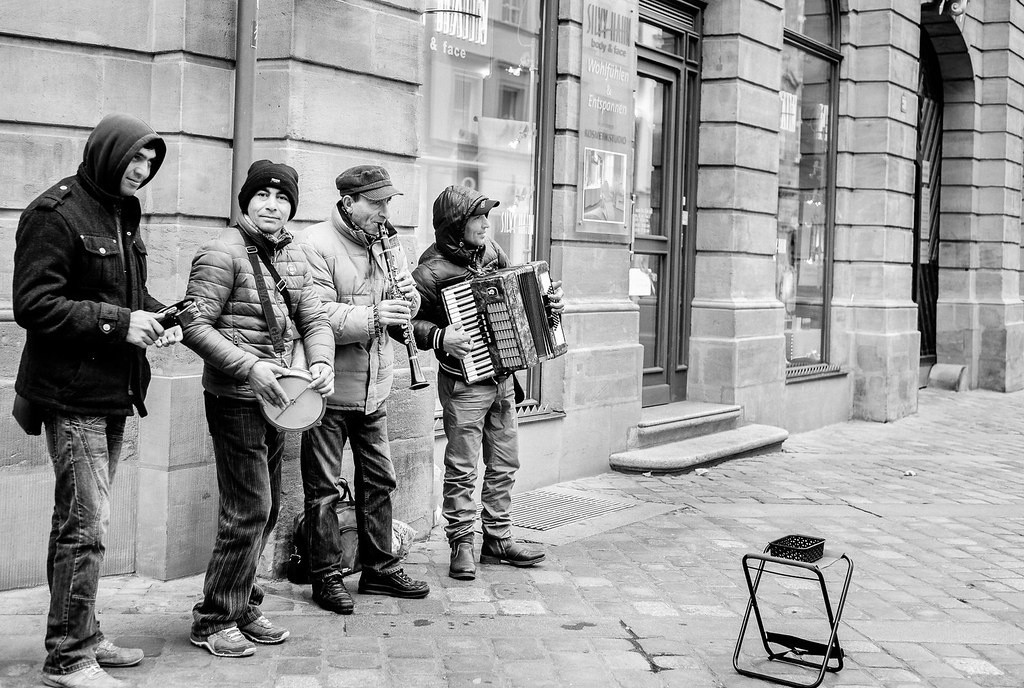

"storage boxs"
[770,535,826,563]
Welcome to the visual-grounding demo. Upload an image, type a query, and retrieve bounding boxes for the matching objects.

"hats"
[335,165,404,201]
[470,199,500,217]
[238,159,299,220]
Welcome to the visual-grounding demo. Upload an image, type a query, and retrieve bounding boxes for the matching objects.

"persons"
[300,166,429,612]
[388,186,565,579]
[182,159,336,657]
[13,114,183,688]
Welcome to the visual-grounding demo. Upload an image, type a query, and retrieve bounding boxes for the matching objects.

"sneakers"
[42,658,120,688]
[357,568,430,599]
[95,639,144,666]
[311,574,354,612]
[239,612,290,643]
[189,622,256,656]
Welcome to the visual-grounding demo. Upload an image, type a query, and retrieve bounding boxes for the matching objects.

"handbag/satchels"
[286,476,363,584]
[391,517,417,561]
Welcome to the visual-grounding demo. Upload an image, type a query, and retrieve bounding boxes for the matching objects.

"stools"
[733,543,853,688]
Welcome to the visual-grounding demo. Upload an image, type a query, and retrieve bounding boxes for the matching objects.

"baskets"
[769,534,825,563]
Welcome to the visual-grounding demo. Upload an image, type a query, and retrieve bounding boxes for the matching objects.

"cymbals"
[155,297,201,336]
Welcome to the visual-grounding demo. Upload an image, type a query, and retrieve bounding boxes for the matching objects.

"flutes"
[377,222,430,391]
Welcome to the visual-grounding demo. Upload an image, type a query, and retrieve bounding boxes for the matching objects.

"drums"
[259,338,327,433]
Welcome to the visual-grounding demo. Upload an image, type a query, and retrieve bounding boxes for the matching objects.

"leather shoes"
[449,541,476,578]
[479,534,546,566]
